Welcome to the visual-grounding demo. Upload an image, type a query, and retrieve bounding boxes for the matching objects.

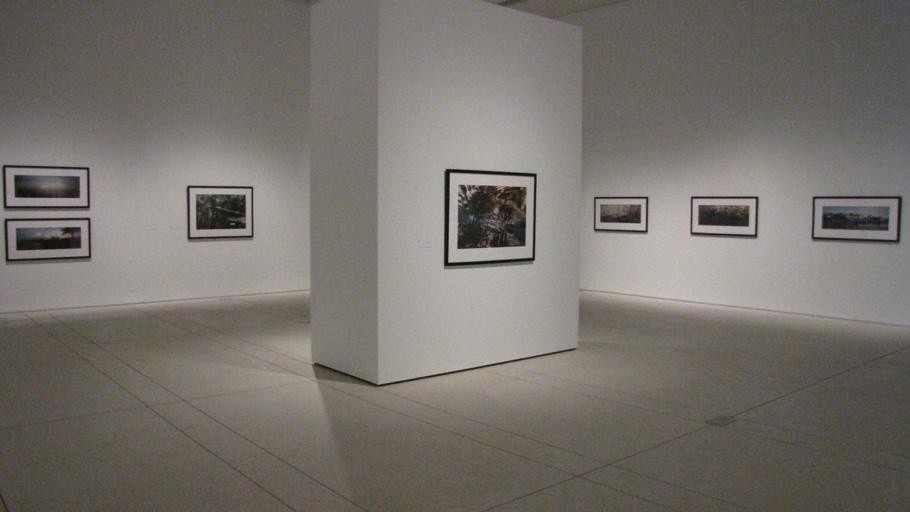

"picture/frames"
[2,164,92,262]
[811,196,901,242]
[594,196,648,232]
[186,185,254,239]
[690,196,758,237]
[444,169,537,266]
